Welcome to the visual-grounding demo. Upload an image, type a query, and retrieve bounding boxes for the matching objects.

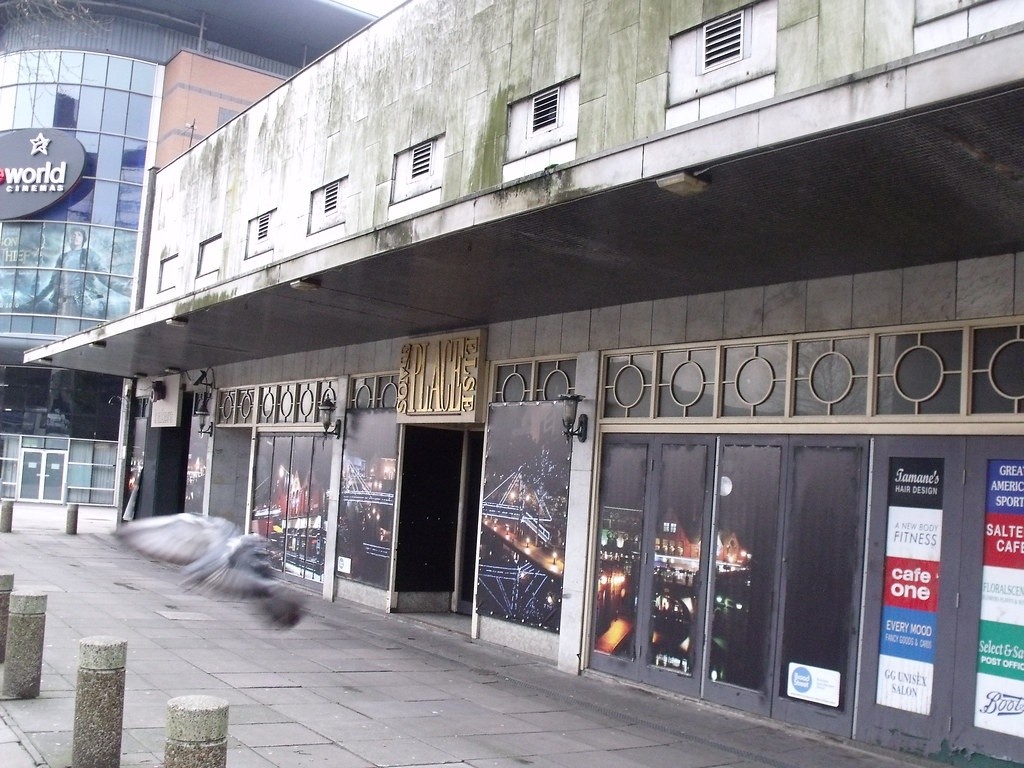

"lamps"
[557,389,589,445]
[319,394,341,440]
[195,401,214,437]
